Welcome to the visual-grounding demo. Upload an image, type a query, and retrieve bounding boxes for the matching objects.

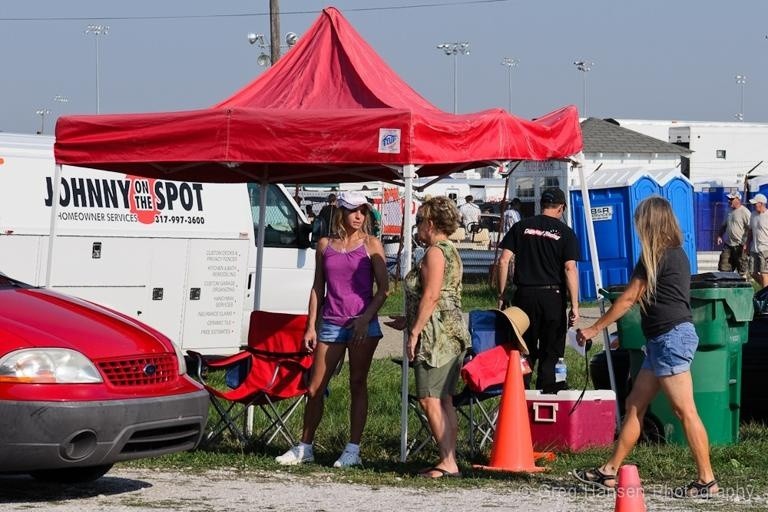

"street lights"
[34,104,52,133]
[437,42,471,116]
[500,55,520,114]
[572,59,596,120]
[85,23,110,115]
[246,32,301,72]
[53,94,72,114]
[731,74,749,121]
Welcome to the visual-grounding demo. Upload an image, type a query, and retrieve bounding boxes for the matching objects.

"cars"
[0,271,214,489]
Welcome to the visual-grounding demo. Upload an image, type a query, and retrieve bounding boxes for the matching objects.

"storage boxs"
[525,388,617,455]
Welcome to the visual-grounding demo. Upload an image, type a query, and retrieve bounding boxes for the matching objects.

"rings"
[359,336,363,340]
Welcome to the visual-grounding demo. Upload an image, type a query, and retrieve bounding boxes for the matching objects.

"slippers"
[414,468,463,479]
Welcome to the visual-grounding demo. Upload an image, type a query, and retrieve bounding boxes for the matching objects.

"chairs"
[389,307,514,467]
[183,310,346,460]
[470,227,490,242]
[446,226,466,243]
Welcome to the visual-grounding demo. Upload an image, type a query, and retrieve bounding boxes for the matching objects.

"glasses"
[416,216,423,226]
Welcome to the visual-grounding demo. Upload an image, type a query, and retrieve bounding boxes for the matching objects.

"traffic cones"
[613,465,647,512]
[471,349,552,474]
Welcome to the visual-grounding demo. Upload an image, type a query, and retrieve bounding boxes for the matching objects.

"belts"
[523,285,560,290]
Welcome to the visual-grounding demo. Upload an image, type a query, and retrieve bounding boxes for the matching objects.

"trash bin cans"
[740,285,768,426]
[598,272,755,450]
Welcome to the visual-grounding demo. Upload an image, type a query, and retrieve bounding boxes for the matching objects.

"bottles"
[555,357,567,384]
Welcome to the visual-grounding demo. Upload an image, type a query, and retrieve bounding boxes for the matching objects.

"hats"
[336,191,373,211]
[489,306,530,355]
[748,194,767,205]
[541,188,567,206]
[725,191,742,199]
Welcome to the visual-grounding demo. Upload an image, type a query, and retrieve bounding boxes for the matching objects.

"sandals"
[672,480,719,499]
[573,467,617,492]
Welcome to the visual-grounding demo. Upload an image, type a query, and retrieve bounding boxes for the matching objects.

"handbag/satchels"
[461,340,532,392]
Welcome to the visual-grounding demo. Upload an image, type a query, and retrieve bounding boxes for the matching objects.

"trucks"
[0,133,328,364]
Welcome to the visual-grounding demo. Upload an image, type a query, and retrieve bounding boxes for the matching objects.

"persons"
[306,204,316,223]
[714,190,751,276]
[458,195,481,233]
[501,197,521,236]
[402,194,473,479]
[319,194,338,235]
[497,186,579,394]
[367,198,381,239]
[274,190,389,471]
[573,195,719,500]
[743,193,768,289]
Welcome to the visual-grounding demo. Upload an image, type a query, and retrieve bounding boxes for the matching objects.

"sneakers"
[275,446,315,466]
[333,449,362,468]
